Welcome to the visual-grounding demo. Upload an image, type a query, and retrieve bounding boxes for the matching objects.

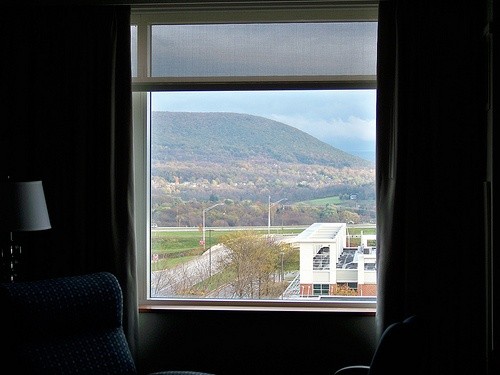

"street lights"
[202,203,225,253]
[268,194,288,236]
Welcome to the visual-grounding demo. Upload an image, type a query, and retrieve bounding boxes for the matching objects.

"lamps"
[0,178,52,283]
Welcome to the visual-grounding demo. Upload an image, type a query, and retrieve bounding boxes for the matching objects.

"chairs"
[331,310,478,375]
[0,272,214,375]
